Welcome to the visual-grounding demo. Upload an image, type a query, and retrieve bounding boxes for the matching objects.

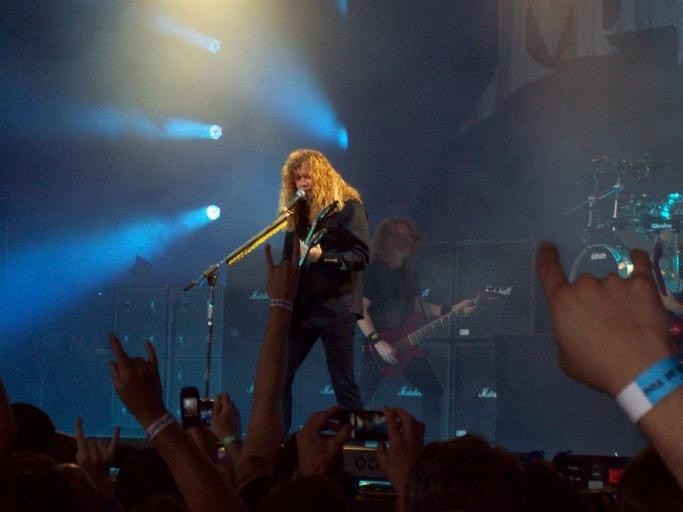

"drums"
[641,192,683,242]
[614,194,656,252]
[567,243,674,285]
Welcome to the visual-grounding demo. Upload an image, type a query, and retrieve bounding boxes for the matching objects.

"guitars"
[366,283,517,378]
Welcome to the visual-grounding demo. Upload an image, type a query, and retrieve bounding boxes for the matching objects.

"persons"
[0,332,682,511]
[276,148,369,436]
[535,242,682,482]
[352,210,477,445]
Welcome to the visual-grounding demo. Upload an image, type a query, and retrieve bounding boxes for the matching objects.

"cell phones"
[180,386,203,430]
[107,465,120,487]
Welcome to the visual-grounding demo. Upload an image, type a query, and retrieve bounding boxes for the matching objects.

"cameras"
[200,399,214,416]
[338,409,389,443]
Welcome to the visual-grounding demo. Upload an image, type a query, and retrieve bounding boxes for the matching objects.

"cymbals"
[618,160,679,175]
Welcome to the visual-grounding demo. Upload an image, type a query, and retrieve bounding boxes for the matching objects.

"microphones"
[403,256,422,296]
[280,189,306,215]
